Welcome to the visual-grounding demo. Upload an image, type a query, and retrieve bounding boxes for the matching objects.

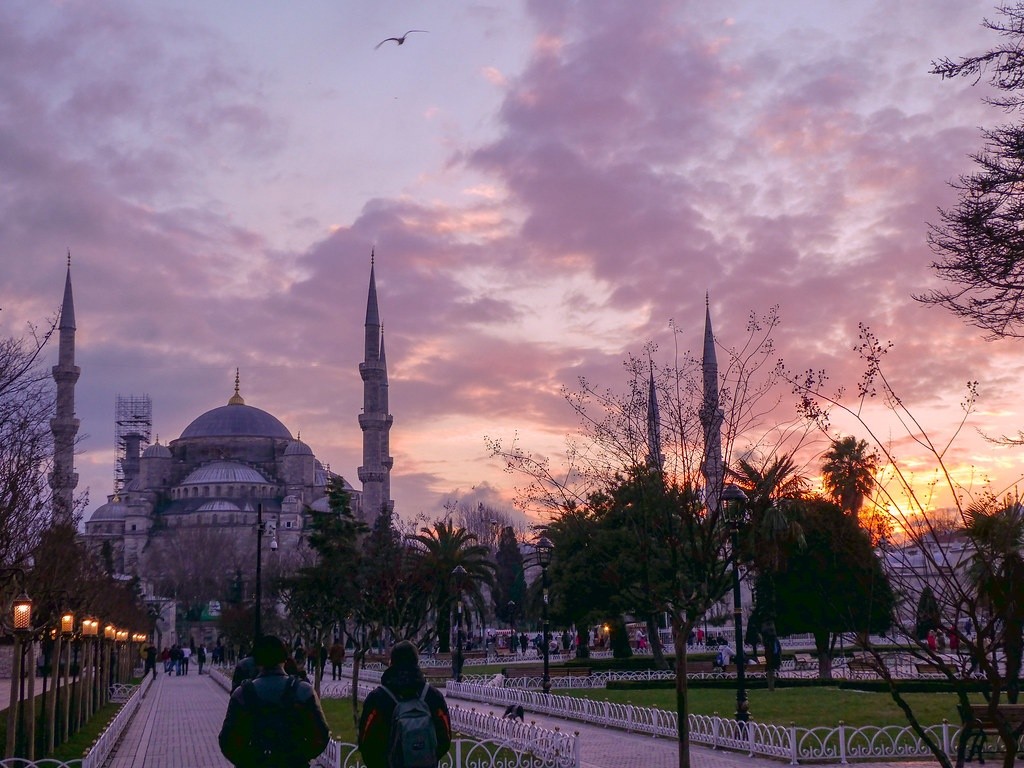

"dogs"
[489,673,505,688]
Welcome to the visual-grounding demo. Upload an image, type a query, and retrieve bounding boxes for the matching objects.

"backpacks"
[240,675,302,768]
[712,646,728,667]
[377,682,439,768]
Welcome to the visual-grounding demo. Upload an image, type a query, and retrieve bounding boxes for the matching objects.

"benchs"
[847,661,879,680]
[502,668,592,688]
[724,663,779,678]
[957,703,1024,761]
[674,662,719,677]
[916,664,961,680]
[794,653,820,670]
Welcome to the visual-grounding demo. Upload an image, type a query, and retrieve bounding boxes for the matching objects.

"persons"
[218,635,331,768]
[329,637,346,681]
[197,644,207,676]
[162,642,191,676]
[927,618,990,674]
[356,641,451,768]
[713,638,737,679]
[452,643,462,680]
[143,645,158,680]
[484,627,725,662]
[211,646,217,666]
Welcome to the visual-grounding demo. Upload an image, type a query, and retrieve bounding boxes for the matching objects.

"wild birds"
[502,703,524,723]
[373,29,429,51]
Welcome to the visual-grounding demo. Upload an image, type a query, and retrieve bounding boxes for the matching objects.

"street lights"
[451,565,467,684]
[508,601,516,653]
[534,535,555,705]
[4,589,32,768]
[719,482,751,743]
[50,608,147,749]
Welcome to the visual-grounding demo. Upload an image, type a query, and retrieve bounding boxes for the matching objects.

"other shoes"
[199,673,203,674]
[523,652,524,656]
